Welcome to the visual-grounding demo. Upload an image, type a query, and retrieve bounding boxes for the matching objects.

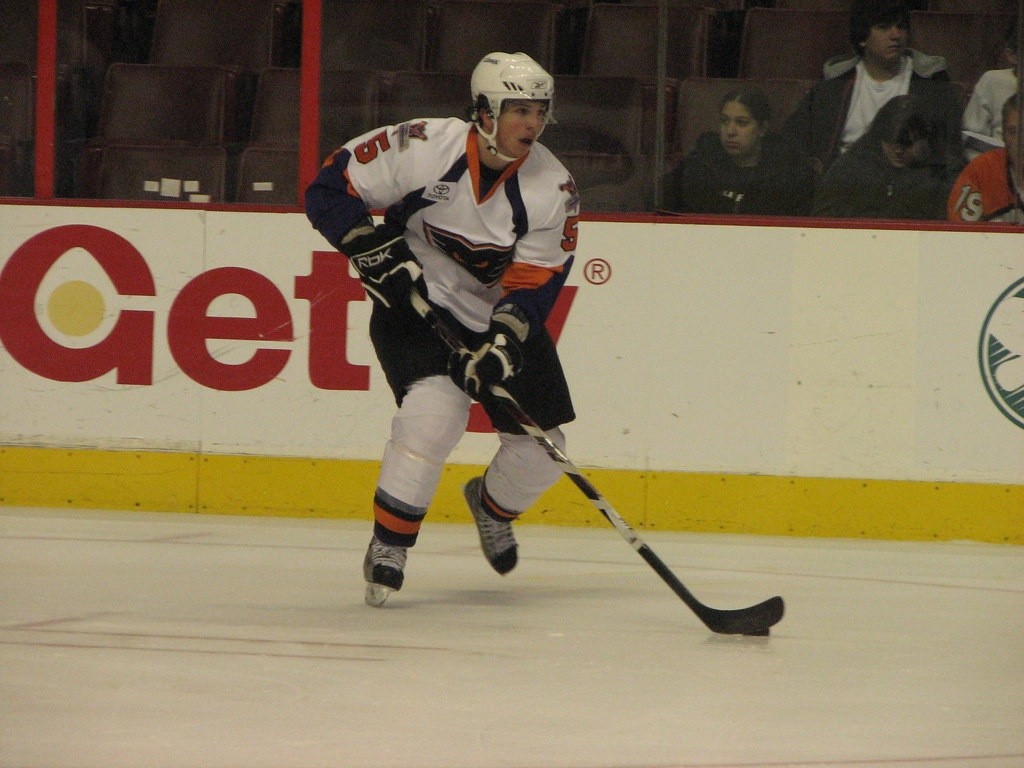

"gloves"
[336,223,427,322]
[448,300,529,402]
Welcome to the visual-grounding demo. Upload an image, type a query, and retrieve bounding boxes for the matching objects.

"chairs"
[1,1,1024,225]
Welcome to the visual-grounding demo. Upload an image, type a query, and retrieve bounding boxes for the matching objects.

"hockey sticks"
[409,287,785,634]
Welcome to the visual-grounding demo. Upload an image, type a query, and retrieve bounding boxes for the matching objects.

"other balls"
[744,628,770,635]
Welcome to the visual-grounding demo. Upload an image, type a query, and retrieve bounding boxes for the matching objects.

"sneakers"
[363,534,408,607]
[465,466,518,574]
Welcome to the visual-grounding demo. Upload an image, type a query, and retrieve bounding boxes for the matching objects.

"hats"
[873,94,931,145]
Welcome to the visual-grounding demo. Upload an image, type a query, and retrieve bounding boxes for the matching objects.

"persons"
[304,51,580,591]
[641,0,1024,223]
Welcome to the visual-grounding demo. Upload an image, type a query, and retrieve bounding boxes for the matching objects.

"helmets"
[470,51,555,118]
